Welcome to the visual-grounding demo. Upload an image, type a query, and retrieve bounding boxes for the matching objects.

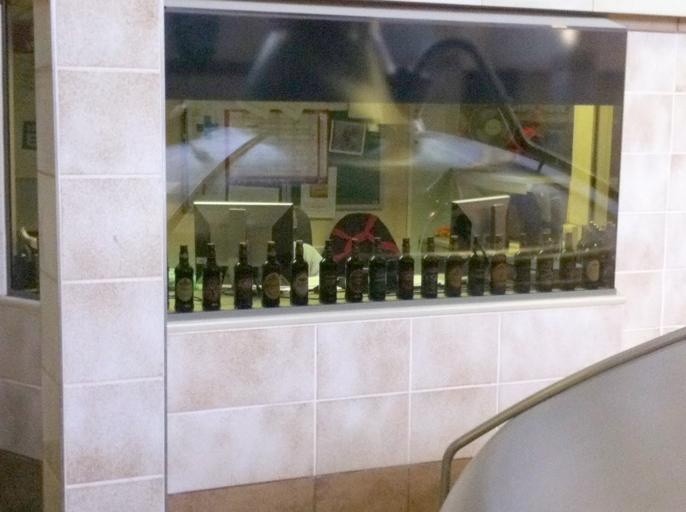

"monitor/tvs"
[193,201,295,284]
[449,194,513,249]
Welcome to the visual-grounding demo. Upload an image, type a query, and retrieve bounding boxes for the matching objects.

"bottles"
[577,220,615,290]
[202,243,220,310]
[344,238,363,302]
[558,232,576,291]
[490,234,506,294]
[420,237,437,298]
[444,235,463,297]
[261,241,280,307]
[233,243,252,309]
[319,239,336,304]
[467,236,486,295]
[174,244,193,312]
[513,233,530,293]
[289,240,308,306]
[367,237,385,301]
[535,231,552,292]
[396,238,414,300]
